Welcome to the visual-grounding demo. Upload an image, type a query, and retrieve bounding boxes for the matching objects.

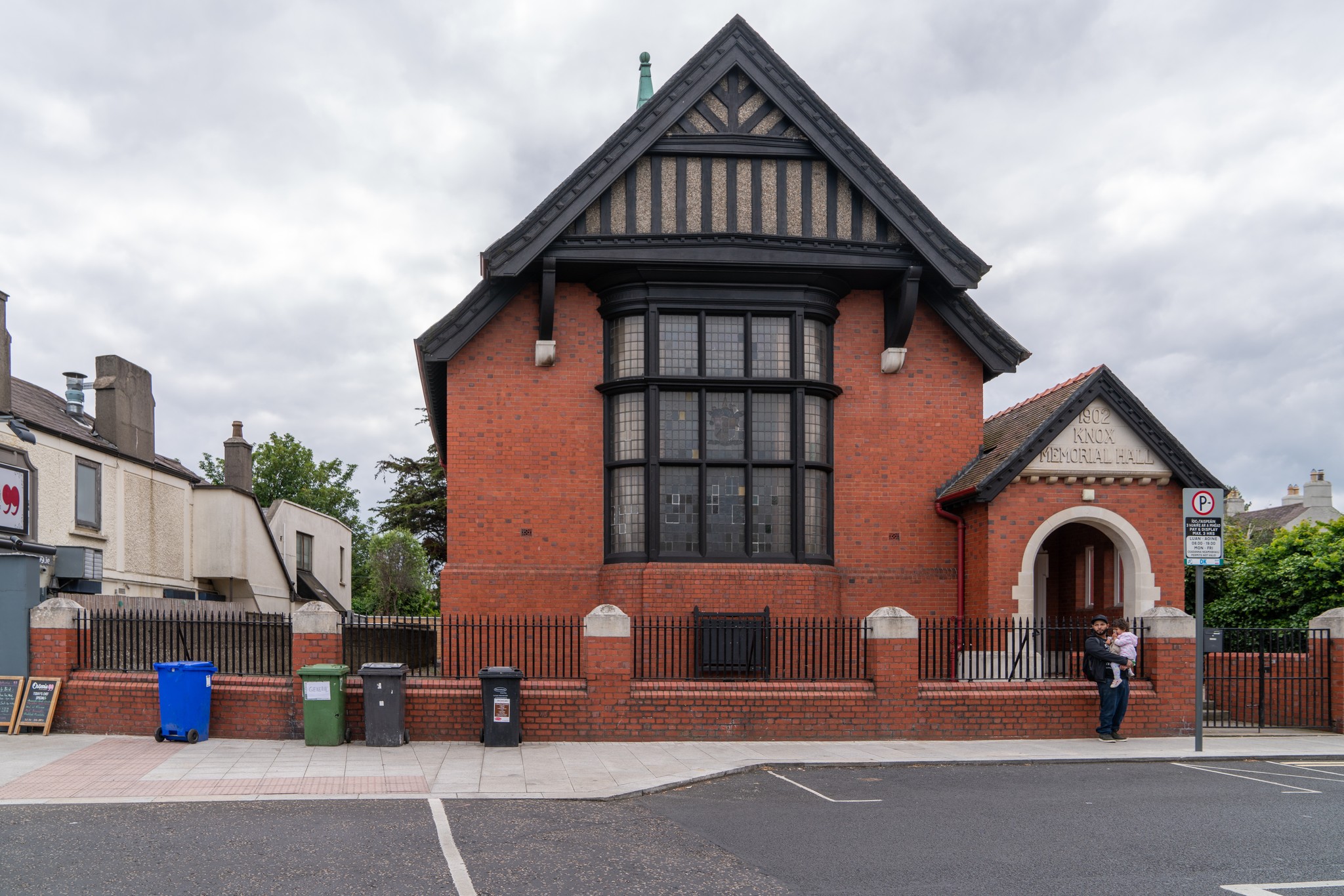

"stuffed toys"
[1110,630,1120,656]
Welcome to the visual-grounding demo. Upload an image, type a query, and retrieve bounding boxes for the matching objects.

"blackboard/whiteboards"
[0,675,25,724]
[18,678,62,726]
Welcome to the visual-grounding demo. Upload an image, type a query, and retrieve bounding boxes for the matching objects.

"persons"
[1105,618,1138,688]
[1085,614,1133,742]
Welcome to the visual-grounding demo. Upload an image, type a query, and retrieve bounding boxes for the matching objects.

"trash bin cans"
[152,661,218,741]
[297,663,350,745]
[478,665,522,747]
[359,662,410,746]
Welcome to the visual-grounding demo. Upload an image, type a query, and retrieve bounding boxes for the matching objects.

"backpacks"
[1082,636,1100,681]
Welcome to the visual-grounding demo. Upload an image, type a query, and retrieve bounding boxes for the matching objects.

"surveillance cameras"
[40,566,47,573]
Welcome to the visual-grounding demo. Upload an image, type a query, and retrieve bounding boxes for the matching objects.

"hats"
[1091,615,1108,624]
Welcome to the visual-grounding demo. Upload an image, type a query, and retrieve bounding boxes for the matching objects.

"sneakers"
[1098,733,1116,742]
[1110,678,1122,688]
[1111,732,1128,742]
[1128,672,1135,678]
